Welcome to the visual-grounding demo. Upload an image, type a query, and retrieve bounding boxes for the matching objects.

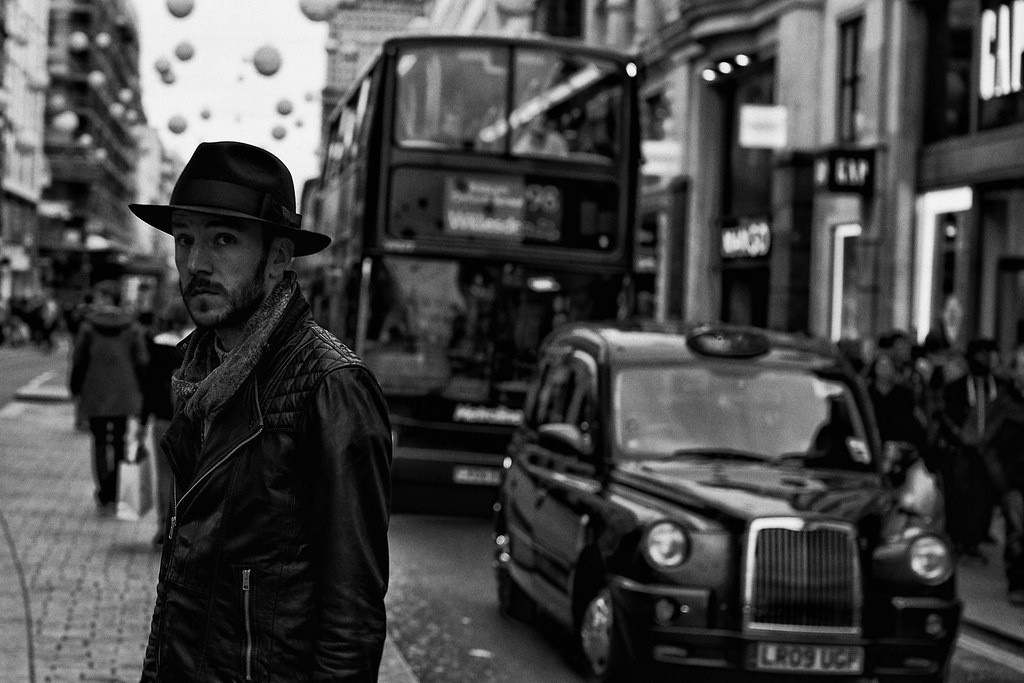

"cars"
[492,320,963,683]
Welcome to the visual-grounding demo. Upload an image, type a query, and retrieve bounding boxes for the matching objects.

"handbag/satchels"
[116,448,154,522]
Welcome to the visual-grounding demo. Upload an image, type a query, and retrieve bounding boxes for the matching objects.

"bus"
[296,32,645,486]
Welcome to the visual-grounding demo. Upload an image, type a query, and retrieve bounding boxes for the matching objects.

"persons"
[382,284,410,342]
[7,282,197,546]
[834,329,1023,607]
[128,139,393,683]
[433,91,572,160]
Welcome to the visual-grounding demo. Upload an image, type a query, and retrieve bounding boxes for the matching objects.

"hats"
[128,141,332,258]
